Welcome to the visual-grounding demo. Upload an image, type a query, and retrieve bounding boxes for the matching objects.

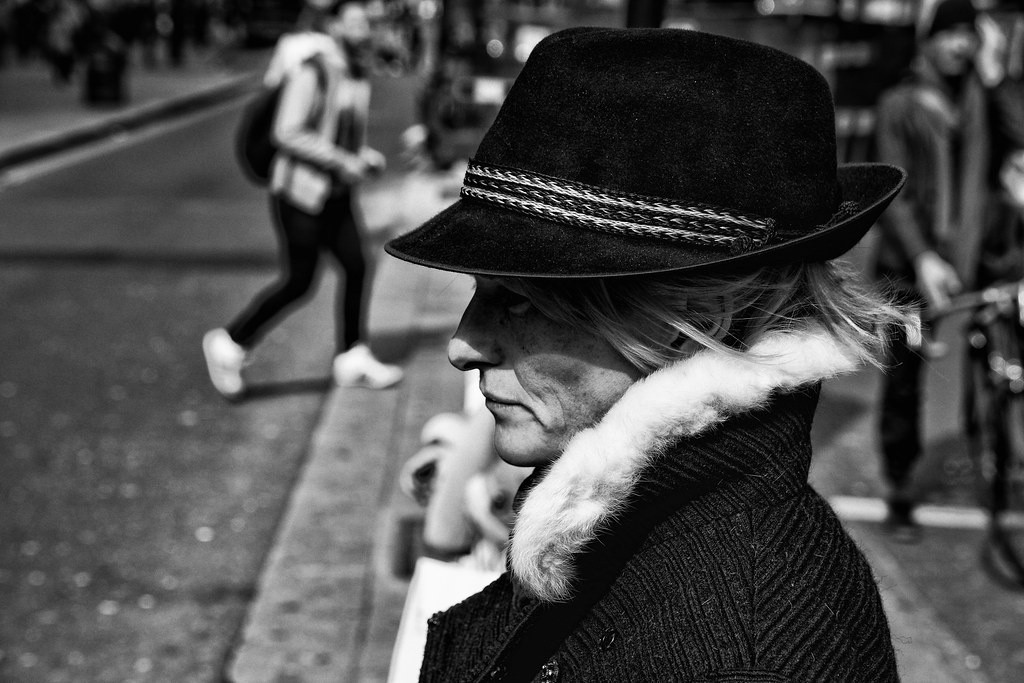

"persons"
[874,0,1024,592]
[386,28,907,683]
[202,0,402,401]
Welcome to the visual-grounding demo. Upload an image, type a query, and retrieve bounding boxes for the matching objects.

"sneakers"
[203,328,247,396]
[332,344,404,389]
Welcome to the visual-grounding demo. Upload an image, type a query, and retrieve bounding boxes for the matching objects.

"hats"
[383,26,907,280]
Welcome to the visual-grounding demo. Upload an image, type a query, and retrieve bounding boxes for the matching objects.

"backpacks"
[232,57,329,187]
